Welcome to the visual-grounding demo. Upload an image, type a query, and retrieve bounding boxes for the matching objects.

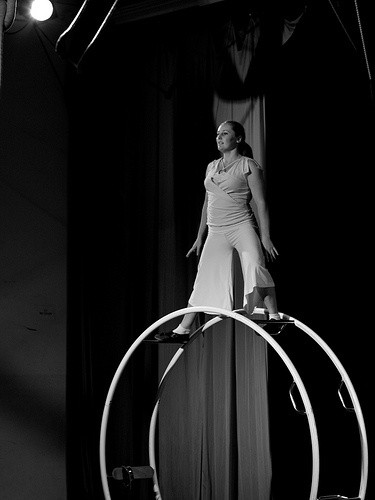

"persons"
[149,120,285,344]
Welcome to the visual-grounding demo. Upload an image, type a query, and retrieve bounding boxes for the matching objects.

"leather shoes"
[143,331,191,343]
[257,320,283,334]
